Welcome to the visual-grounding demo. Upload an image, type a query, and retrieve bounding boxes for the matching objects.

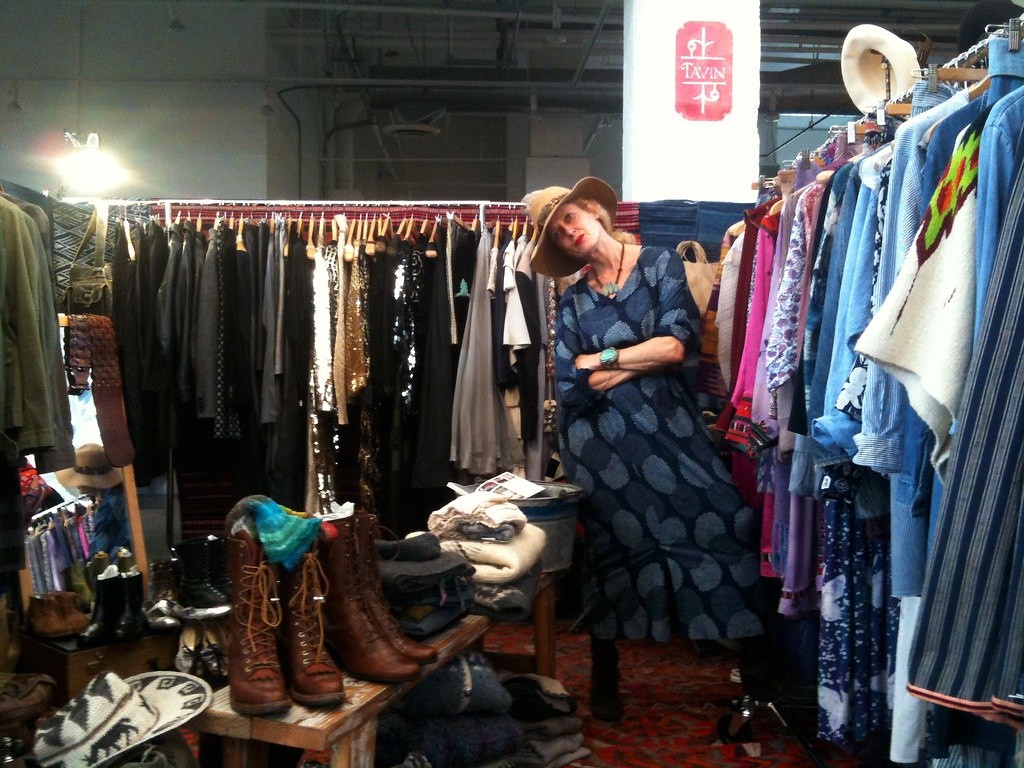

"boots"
[233,512,435,714]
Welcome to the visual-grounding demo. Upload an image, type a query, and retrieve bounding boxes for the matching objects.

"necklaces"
[591,241,624,298]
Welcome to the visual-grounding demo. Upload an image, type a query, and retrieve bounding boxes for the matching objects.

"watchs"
[599,347,620,370]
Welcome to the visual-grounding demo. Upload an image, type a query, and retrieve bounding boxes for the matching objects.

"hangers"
[106,18,1024,270]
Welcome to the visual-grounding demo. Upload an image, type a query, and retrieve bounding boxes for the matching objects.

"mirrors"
[54,312,150,617]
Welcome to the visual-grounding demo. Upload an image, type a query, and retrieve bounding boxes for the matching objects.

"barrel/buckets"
[456,478,583,574]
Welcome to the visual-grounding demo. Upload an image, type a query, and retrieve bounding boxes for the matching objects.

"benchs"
[178,612,494,768]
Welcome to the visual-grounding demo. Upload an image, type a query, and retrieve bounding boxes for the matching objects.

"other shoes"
[4,536,228,725]
[592,639,625,720]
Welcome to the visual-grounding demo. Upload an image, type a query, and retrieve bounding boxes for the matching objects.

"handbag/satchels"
[70,207,113,313]
[677,240,719,315]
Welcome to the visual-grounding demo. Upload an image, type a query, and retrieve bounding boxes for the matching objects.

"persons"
[75,449,134,561]
[533,188,778,724]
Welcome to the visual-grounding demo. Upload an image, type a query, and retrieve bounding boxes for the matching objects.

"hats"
[55,443,125,490]
[524,176,619,280]
[28,669,209,767]
[840,24,922,112]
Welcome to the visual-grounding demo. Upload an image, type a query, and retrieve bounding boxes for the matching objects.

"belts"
[65,313,134,470]
